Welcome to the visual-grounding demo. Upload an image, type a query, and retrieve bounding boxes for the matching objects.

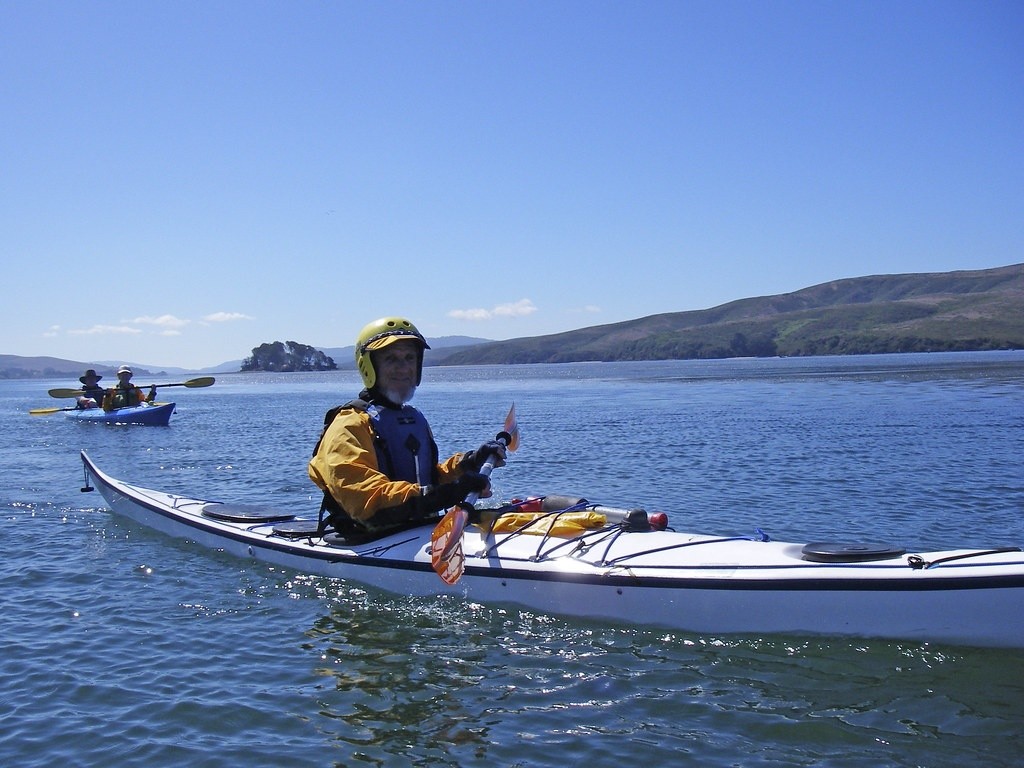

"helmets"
[355,317,431,389]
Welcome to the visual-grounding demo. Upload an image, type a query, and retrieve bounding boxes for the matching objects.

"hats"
[116,365,133,378]
[79,370,102,384]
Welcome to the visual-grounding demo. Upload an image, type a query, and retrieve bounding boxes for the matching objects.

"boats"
[80,449,1024,651]
[64,402,176,424]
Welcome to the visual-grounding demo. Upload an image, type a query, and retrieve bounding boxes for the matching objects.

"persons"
[309,316,507,546]
[75,370,104,406]
[101,365,157,410]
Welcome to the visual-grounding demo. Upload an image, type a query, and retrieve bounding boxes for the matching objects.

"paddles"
[48,376,216,399]
[30,402,173,416]
[431,403,521,584]
